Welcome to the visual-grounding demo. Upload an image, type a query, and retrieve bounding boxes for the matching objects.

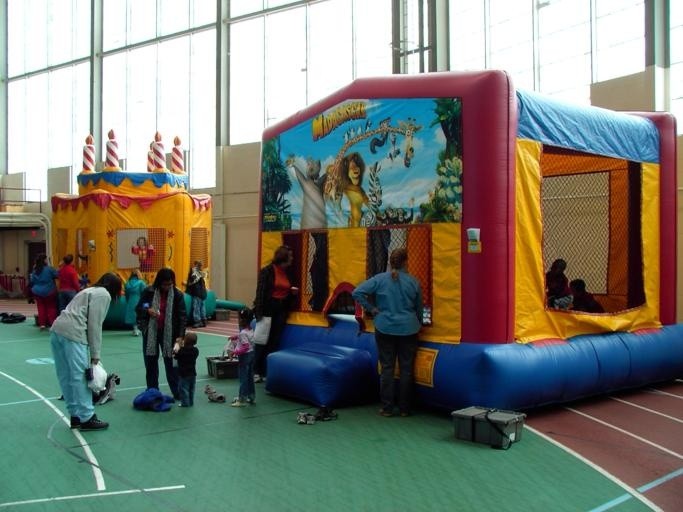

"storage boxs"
[451,407,525,448]
[207,355,238,378]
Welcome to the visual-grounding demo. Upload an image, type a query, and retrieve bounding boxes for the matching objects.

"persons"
[135,267,187,400]
[57,254,81,315]
[185,260,208,328]
[172,332,199,408]
[545,258,569,309]
[30,255,57,329]
[227,307,257,408]
[569,279,605,315]
[351,248,424,419]
[49,271,122,429]
[124,272,146,336]
[252,245,300,383]
[131,237,156,272]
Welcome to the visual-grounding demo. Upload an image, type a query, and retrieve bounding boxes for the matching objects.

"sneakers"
[70,416,80,427]
[210,393,225,403]
[254,374,262,382]
[202,320,207,326]
[299,413,305,423]
[317,411,340,420]
[307,415,313,424]
[40,325,44,329]
[205,384,214,393]
[134,330,140,336]
[81,414,109,431]
[379,409,390,416]
[193,322,202,327]
[401,409,414,417]
